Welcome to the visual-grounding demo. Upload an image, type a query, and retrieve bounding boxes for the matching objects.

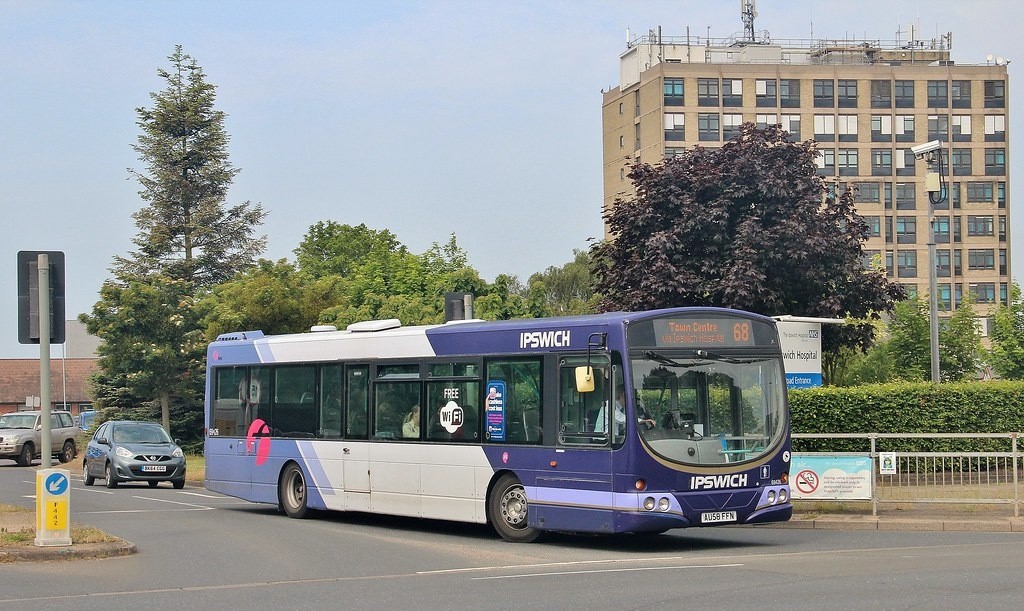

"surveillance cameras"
[911,139,941,155]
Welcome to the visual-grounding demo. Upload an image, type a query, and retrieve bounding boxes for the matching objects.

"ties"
[618,408,624,435]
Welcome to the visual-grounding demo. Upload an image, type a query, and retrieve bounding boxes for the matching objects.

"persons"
[377,383,480,443]
[589,385,656,442]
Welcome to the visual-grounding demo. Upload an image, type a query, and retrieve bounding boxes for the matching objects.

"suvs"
[0,409,79,467]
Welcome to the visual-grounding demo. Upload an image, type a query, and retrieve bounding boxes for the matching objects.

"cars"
[83,421,186,490]
[79,411,100,431]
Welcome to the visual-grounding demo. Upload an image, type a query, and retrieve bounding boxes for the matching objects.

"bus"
[203,306,792,542]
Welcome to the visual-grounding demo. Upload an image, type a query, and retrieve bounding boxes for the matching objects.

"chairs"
[523,400,540,442]
[325,394,342,430]
[350,408,368,435]
[300,391,315,403]
[461,405,477,439]
